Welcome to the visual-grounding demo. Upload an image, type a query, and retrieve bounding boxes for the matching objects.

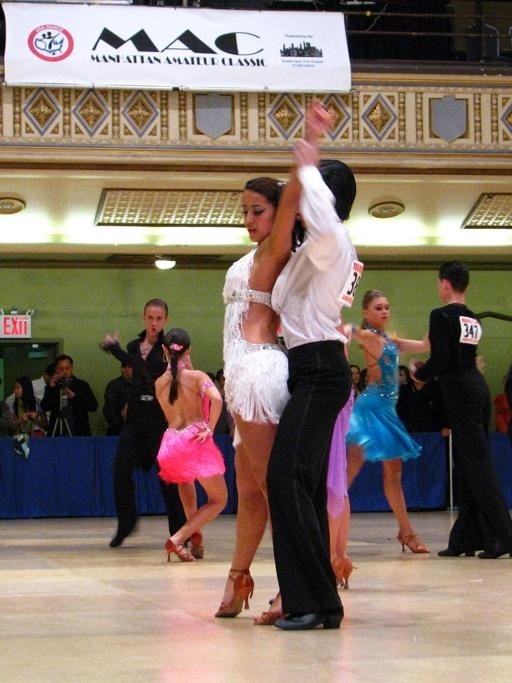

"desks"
[0,432,446,518]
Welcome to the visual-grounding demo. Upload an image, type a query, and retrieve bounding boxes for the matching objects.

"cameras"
[53,377,67,396]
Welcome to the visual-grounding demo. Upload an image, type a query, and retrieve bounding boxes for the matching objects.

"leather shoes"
[273,606,346,631]
[437,544,476,557]
[478,544,512,560]
[108,523,135,548]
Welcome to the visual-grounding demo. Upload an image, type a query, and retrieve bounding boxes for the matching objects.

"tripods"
[50,413,73,438]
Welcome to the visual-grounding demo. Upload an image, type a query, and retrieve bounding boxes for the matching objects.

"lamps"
[155,257,176,270]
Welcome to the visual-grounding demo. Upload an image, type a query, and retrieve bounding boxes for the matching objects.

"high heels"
[253,586,282,625]
[215,565,254,620]
[331,553,345,588]
[396,531,430,555]
[189,531,206,560]
[164,538,195,563]
[343,557,354,591]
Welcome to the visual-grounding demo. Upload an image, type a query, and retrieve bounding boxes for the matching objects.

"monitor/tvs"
[0,337,64,399]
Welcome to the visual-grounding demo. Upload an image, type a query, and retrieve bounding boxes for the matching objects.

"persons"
[215,98,329,626]
[0,259,512,588]
[272,139,365,630]
